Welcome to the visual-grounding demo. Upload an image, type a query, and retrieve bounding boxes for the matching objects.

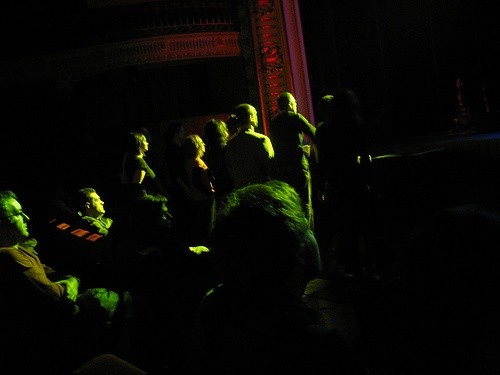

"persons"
[0,190,129,322]
[72,92,396,290]
[190,180,370,375]
